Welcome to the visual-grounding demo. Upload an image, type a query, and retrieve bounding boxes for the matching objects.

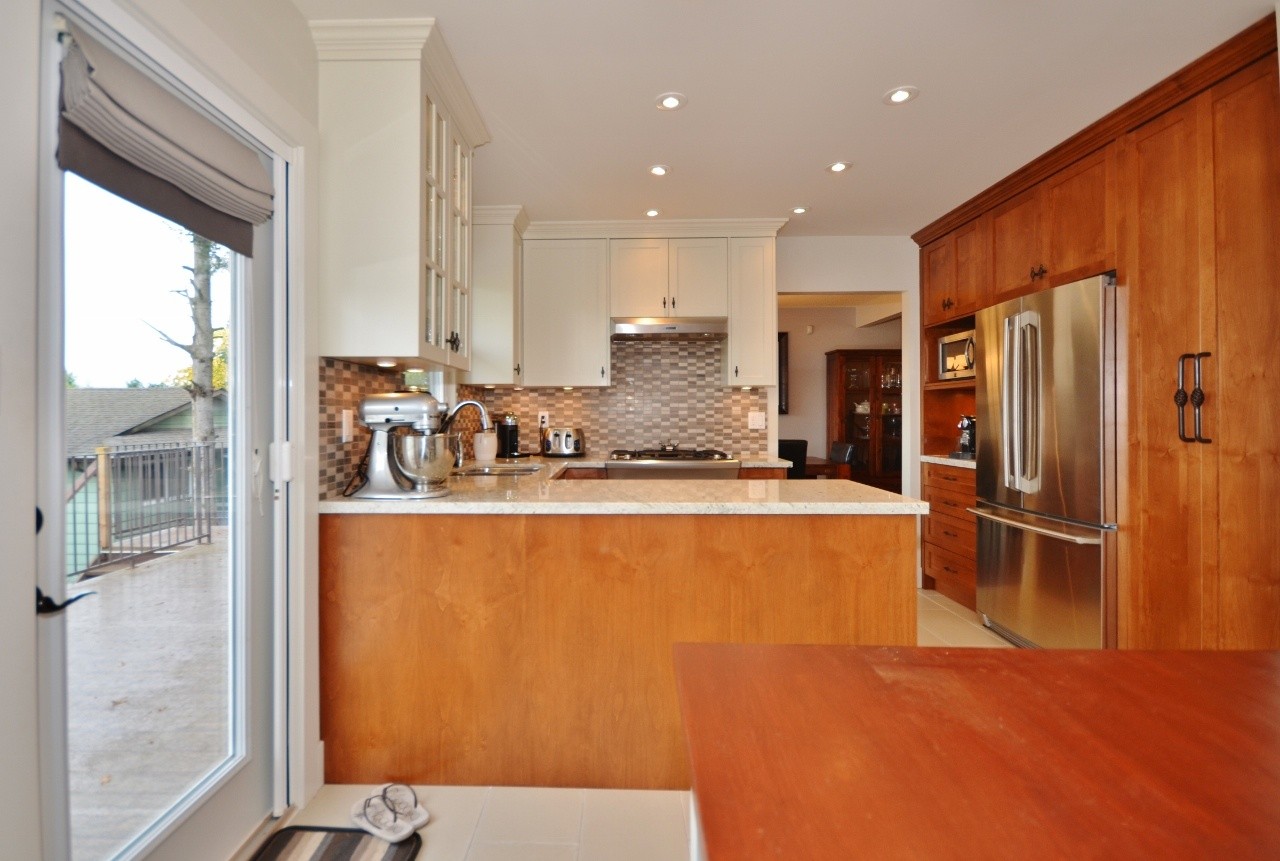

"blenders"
[349,391,460,500]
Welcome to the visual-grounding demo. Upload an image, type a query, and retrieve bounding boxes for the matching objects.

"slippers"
[352,794,415,843]
[375,783,430,829]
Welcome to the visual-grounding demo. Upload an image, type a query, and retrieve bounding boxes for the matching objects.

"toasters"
[543,427,586,458]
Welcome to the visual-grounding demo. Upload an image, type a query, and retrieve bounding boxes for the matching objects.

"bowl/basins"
[392,434,457,491]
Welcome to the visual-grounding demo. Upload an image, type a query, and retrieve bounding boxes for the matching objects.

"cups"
[473,433,498,461]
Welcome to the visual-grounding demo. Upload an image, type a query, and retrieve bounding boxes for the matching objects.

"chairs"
[829,442,853,463]
[779,440,807,479]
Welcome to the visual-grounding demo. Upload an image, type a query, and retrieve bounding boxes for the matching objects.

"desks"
[806,456,851,479]
[672,643,1280,861]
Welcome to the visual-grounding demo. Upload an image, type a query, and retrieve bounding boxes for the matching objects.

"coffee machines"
[958,414,975,454]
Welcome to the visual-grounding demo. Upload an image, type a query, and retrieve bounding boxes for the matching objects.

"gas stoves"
[609,450,732,461]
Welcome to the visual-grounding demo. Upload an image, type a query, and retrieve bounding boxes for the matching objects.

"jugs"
[853,399,870,413]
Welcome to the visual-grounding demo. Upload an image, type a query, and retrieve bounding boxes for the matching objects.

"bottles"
[540,416,547,447]
[881,402,901,415]
[845,365,901,389]
[496,412,518,458]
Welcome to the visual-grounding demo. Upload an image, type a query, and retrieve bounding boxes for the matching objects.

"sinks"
[448,467,542,476]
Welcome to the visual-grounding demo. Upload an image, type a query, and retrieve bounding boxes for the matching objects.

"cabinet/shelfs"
[609,220,728,324]
[738,468,787,479]
[976,111,1116,311]
[722,219,789,386]
[457,206,531,391]
[912,188,976,610]
[563,468,607,479]
[307,18,490,372]
[825,349,901,495]
[1116,12,1280,651]
[523,221,610,387]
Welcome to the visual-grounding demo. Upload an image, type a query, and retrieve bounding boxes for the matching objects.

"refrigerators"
[973,273,1124,651]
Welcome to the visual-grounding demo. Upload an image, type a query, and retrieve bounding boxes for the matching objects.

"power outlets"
[538,411,549,429]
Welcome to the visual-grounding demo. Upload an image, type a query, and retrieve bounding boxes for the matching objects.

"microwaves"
[937,330,977,381]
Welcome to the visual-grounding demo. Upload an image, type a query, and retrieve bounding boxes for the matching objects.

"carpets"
[250,825,423,860]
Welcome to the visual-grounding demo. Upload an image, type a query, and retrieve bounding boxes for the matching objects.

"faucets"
[439,400,495,433]
